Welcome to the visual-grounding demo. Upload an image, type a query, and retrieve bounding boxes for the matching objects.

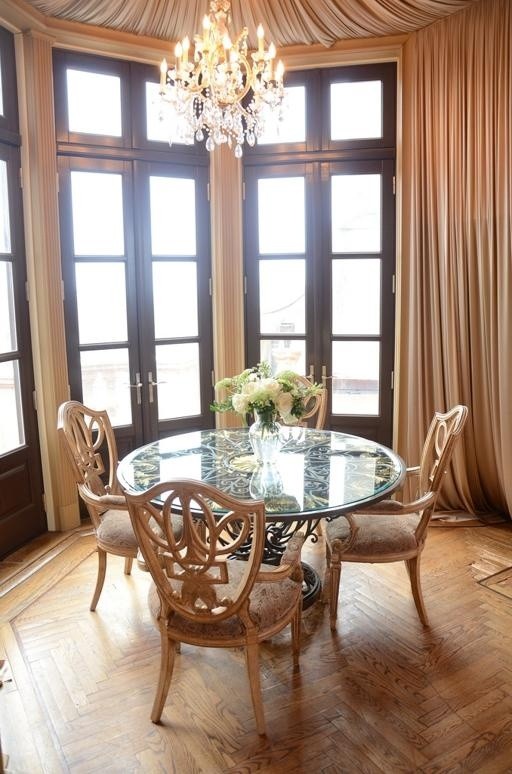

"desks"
[116,425,408,612]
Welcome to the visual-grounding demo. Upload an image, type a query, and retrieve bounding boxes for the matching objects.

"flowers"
[207,359,325,429]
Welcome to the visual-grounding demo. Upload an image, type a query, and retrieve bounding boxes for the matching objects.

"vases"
[247,407,286,465]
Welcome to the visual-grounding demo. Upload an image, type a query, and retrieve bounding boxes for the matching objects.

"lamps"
[158,1,286,160]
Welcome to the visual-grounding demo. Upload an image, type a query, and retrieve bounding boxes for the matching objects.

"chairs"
[120,477,305,736]
[323,403,470,633]
[254,370,329,536]
[57,400,207,613]
